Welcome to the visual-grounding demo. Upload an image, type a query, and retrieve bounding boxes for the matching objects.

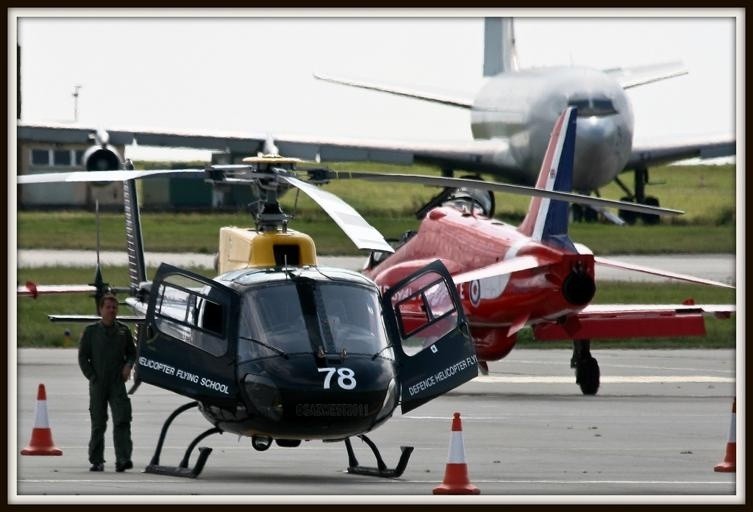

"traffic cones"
[431,411,480,495]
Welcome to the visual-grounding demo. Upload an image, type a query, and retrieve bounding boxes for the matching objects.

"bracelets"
[125,363,131,368]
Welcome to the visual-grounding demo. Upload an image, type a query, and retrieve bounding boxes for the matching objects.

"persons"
[77,296,136,472]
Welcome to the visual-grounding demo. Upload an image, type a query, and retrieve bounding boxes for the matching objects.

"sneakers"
[116,459,132,472]
[90,462,104,471]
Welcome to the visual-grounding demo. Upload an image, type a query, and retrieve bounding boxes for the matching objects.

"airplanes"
[18,17,736,225]
